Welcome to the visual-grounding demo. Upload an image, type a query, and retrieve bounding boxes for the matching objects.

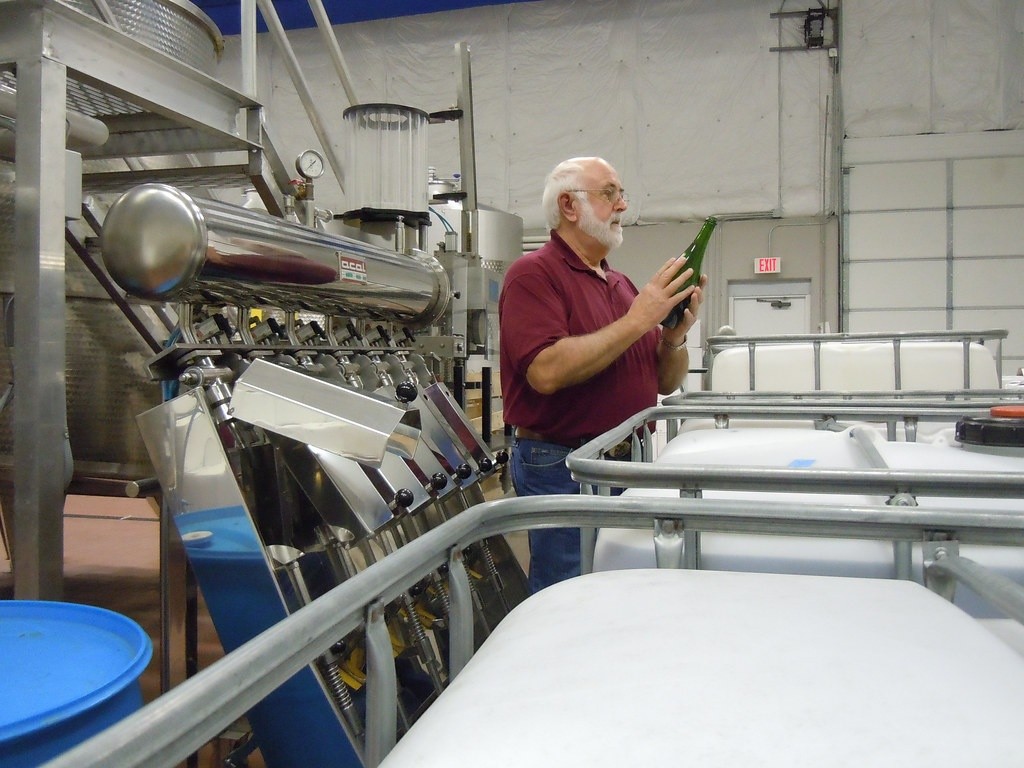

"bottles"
[658,215,717,330]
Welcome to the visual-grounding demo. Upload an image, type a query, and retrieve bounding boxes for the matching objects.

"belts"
[514,426,631,458]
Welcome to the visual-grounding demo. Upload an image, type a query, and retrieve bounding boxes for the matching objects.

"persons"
[498,155,708,597]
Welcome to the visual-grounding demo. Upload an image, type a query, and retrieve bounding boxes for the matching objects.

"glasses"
[565,185,630,205]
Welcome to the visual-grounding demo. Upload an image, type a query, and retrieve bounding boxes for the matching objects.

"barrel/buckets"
[0,599,153,768]
